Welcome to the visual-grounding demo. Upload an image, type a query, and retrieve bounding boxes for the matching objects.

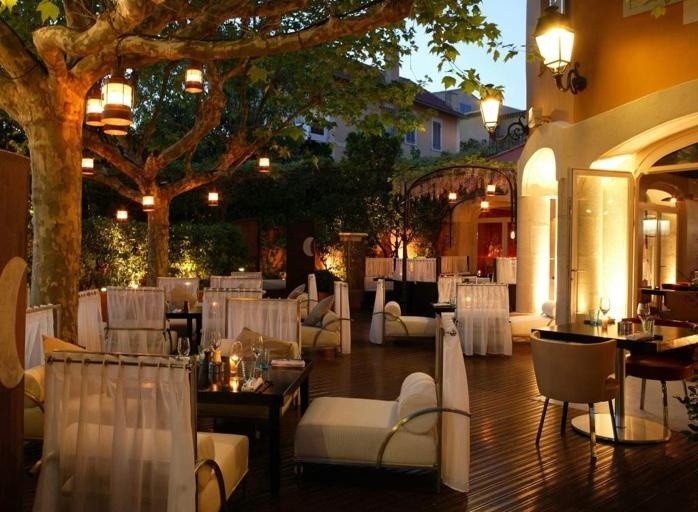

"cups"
[643,320,654,336]
[588,310,598,326]
[259,352,272,383]
[177,337,190,357]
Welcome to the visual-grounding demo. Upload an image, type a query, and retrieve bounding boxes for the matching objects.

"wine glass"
[209,332,221,359]
[599,298,609,327]
[636,303,650,332]
[251,336,263,370]
